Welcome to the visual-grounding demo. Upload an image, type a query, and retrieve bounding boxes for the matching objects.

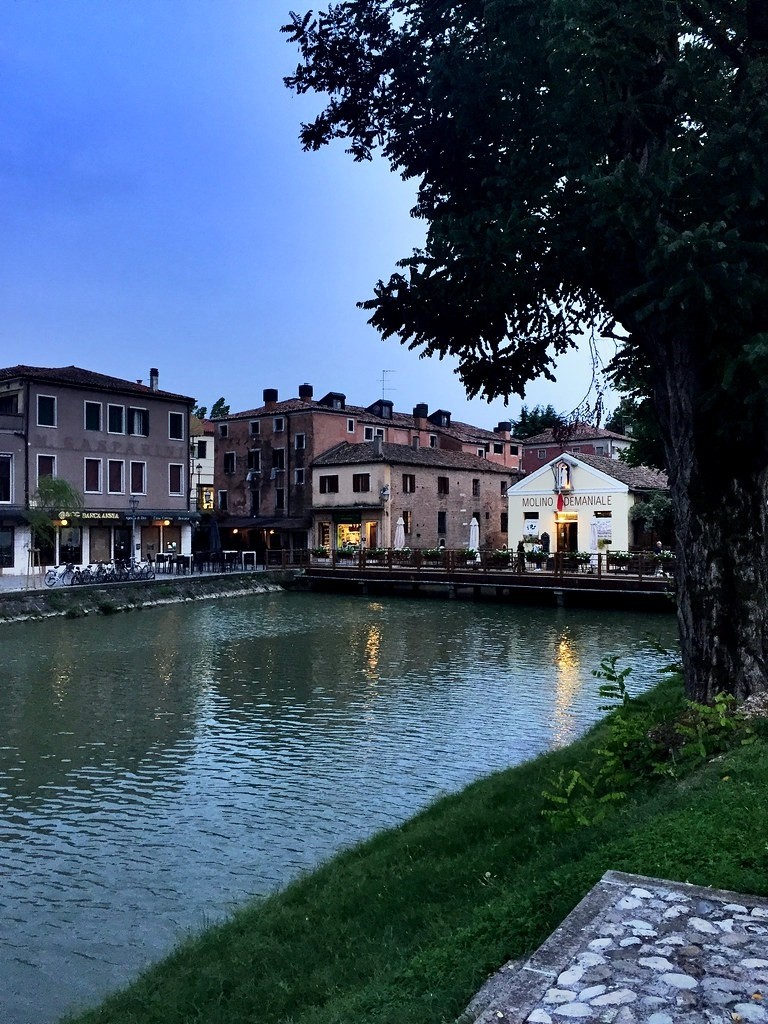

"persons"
[513,541,528,572]
[655,539,665,576]
[532,539,544,570]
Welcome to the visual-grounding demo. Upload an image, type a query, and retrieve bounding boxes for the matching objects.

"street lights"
[196,464,202,502]
[129,496,140,562]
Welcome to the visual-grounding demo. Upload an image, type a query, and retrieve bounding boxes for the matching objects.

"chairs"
[145,553,242,576]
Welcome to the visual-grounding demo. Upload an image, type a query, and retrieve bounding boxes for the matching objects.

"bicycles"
[71,557,155,585]
[44,562,76,587]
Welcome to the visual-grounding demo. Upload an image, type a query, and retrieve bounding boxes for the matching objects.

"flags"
[557,491,565,511]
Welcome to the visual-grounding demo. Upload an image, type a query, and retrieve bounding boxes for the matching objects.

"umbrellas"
[470,517,480,551]
[393,517,405,550]
